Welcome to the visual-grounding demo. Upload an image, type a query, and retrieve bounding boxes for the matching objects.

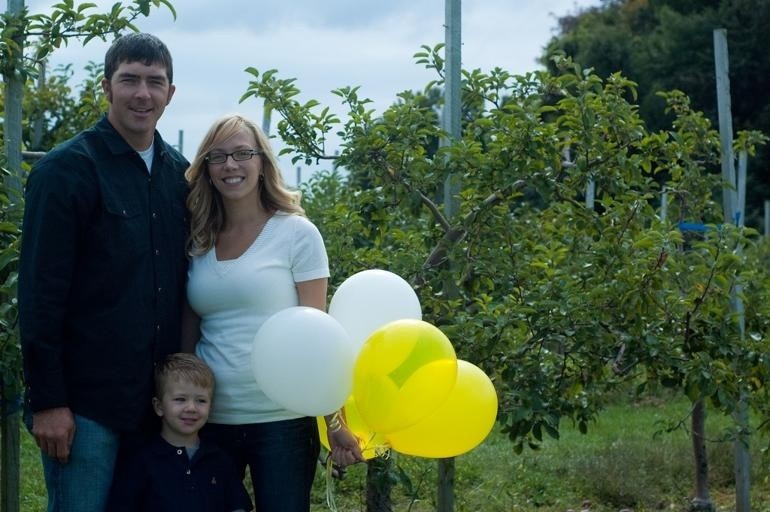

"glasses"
[205,149,263,163]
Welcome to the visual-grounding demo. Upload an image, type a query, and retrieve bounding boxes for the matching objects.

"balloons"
[390,359,498,458]
[355,317,457,434]
[252,306,355,416]
[329,268,422,373]
[316,401,390,462]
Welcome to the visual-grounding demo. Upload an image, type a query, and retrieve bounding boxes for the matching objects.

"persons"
[185,115,358,510]
[18,33,194,511]
[109,354,254,511]
